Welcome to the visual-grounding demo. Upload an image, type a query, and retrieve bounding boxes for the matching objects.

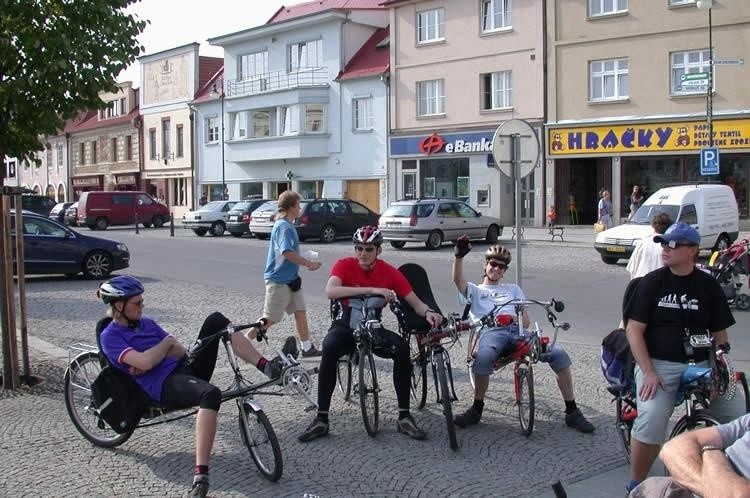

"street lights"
[209,70,227,201]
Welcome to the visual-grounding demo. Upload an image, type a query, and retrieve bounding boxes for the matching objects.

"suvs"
[291,198,383,243]
[248,201,281,239]
[226,198,275,237]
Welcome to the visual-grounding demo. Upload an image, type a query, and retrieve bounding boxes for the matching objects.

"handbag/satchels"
[593,220,606,235]
[286,276,302,293]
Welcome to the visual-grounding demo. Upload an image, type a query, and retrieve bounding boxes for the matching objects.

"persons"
[220,187,229,211]
[597,189,614,230]
[627,185,644,220]
[296,222,445,444]
[96,271,300,497]
[241,189,324,359]
[623,219,750,498]
[158,194,166,205]
[200,192,208,206]
[450,232,597,434]
[547,205,556,235]
[567,189,579,225]
[625,412,750,498]
[618,211,676,331]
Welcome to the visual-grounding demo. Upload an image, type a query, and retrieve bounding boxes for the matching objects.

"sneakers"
[296,416,330,443]
[301,342,322,358]
[395,414,428,441]
[453,402,485,428]
[186,473,211,497]
[565,407,596,435]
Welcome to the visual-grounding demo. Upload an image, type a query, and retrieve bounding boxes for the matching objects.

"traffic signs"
[711,59,744,65]
[676,72,709,91]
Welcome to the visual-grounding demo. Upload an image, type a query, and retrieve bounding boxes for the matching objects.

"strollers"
[701,238,750,311]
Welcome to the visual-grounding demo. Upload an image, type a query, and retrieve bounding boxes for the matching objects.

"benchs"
[511,225,565,241]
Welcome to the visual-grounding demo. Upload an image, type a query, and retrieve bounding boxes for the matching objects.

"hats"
[653,219,701,247]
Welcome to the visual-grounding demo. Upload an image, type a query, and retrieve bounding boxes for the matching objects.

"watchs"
[424,308,435,317]
[701,445,725,454]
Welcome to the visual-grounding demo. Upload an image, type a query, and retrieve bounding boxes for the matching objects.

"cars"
[8,208,130,279]
[376,196,504,250]
[64,201,79,226]
[49,202,73,222]
[182,201,236,237]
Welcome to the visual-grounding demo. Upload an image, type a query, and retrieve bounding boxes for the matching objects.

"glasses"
[127,298,144,306]
[659,240,690,250]
[357,246,376,253]
[488,260,507,270]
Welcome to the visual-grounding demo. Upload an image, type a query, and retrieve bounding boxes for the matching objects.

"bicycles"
[468,292,574,436]
[323,292,409,438]
[384,262,499,451]
[612,326,749,487]
[60,316,321,484]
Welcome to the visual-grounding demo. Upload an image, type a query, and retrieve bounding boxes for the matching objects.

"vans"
[596,184,741,264]
[76,189,171,230]
[10,195,57,218]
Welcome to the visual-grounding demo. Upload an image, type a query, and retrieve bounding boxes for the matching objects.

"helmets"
[485,244,512,265]
[352,224,384,248]
[99,275,145,305]
